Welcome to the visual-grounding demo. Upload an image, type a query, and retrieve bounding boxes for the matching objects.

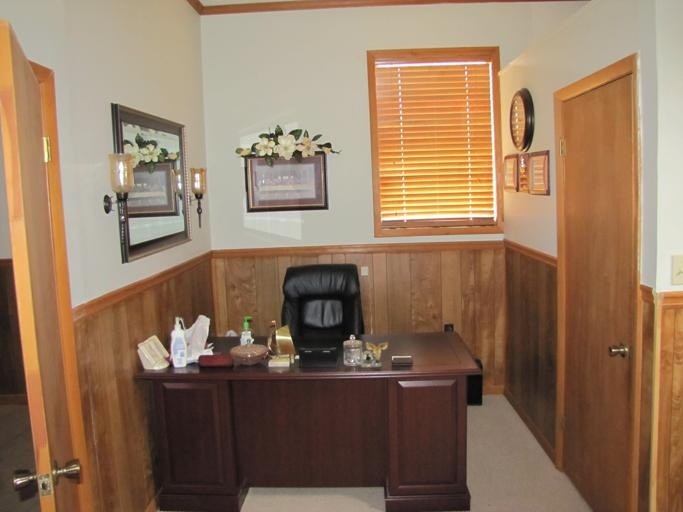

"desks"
[132,331,481,512]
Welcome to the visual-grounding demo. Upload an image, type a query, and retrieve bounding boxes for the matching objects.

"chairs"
[282,264,364,334]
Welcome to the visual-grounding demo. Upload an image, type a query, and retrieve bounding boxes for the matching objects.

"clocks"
[508,88,537,154]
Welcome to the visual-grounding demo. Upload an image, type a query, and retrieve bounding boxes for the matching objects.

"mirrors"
[111,102,195,263]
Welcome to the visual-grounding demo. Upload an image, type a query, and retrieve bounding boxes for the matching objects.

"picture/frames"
[127,161,179,217]
[503,154,521,192]
[244,151,329,212]
[526,149,550,196]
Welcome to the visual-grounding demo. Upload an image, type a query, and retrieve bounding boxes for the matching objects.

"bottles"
[341,334,363,367]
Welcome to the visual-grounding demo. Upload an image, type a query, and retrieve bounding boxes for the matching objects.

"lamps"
[187,165,208,230]
[104,153,134,248]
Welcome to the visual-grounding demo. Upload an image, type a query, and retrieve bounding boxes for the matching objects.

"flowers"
[235,125,342,167]
[121,133,180,169]
